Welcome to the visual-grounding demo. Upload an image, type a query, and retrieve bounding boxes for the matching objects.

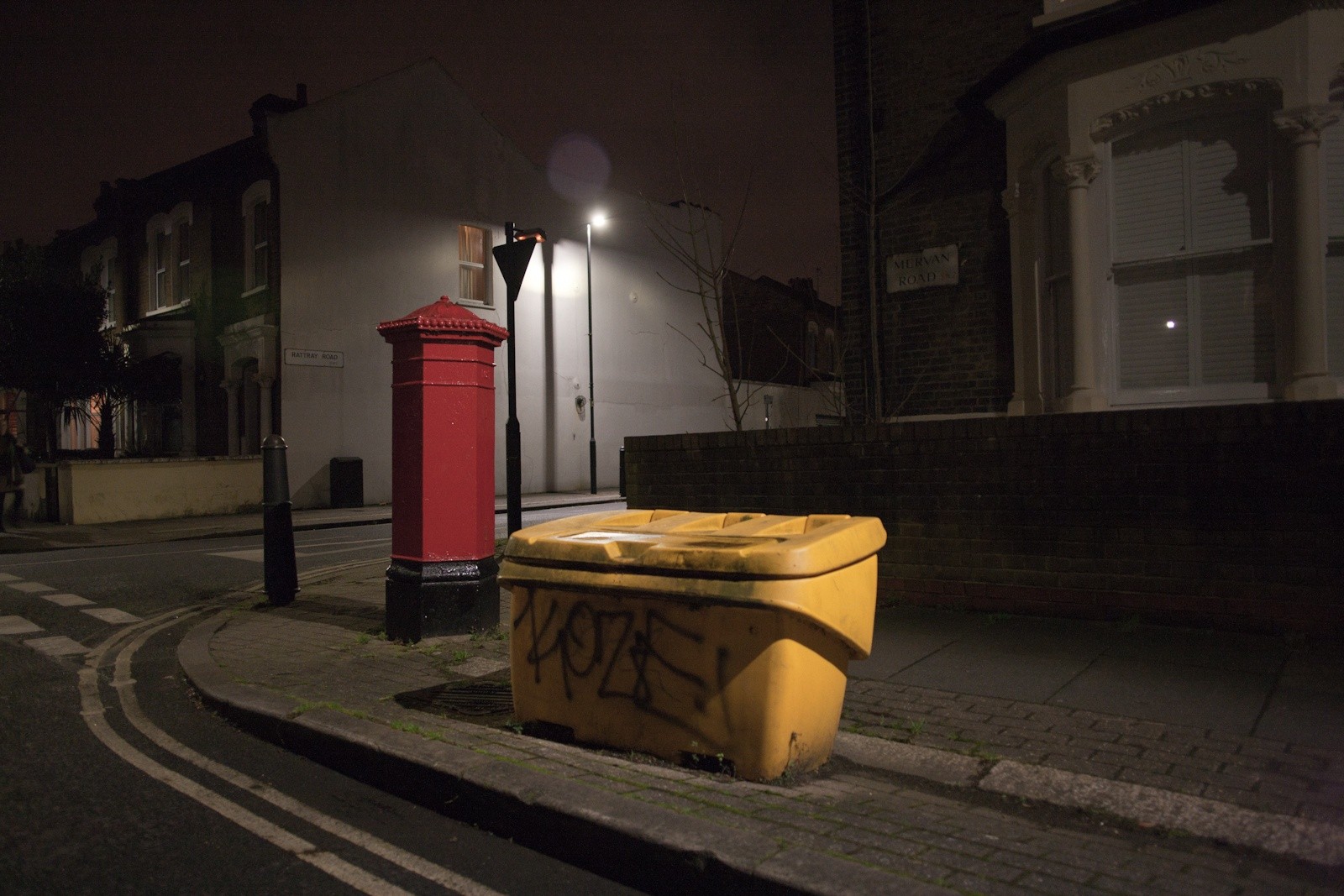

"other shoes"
[9,516,22,528]
[0,521,6,532]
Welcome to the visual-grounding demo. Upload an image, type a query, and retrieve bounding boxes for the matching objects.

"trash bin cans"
[330,456,364,508]
[493,507,888,788]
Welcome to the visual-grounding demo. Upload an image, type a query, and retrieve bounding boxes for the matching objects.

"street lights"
[584,206,614,496]
[503,219,547,537]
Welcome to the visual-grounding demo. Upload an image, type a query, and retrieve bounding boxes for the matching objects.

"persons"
[0,422,34,534]
